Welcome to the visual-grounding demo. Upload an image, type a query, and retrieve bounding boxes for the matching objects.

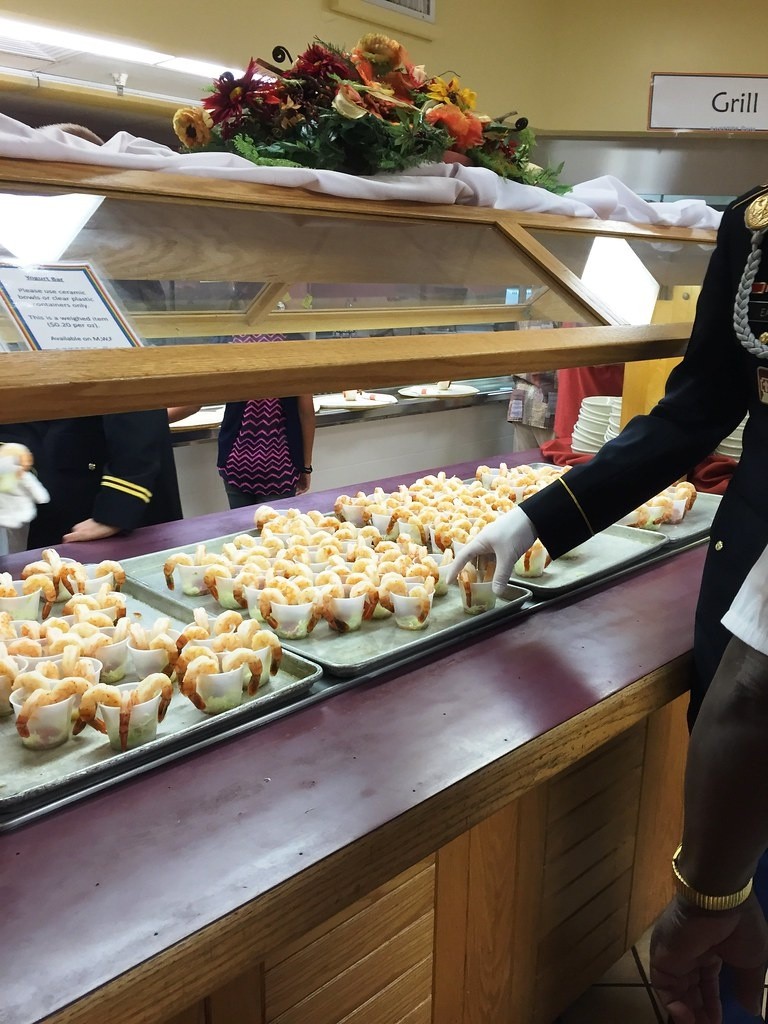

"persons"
[0,408,184,550]
[446,182,768,1024]
[167,333,315,509]
[506,320,559,452]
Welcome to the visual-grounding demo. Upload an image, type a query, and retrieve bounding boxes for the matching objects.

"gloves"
[0,444,50,529]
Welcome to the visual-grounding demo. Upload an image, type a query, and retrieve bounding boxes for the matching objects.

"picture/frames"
[327,1,444,42]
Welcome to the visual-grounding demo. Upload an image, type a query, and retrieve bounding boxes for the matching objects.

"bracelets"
[304,465,313,474]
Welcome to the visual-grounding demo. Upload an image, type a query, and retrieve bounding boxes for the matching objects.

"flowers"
[169,38,572,197]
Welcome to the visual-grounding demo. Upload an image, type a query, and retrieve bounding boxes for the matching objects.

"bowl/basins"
[713,410,750,463]
[570,396,622,457]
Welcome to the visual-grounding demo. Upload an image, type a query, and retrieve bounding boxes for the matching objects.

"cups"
[0,468,687,753]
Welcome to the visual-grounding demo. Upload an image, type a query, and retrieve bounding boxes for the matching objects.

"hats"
[445,504,538,594]
